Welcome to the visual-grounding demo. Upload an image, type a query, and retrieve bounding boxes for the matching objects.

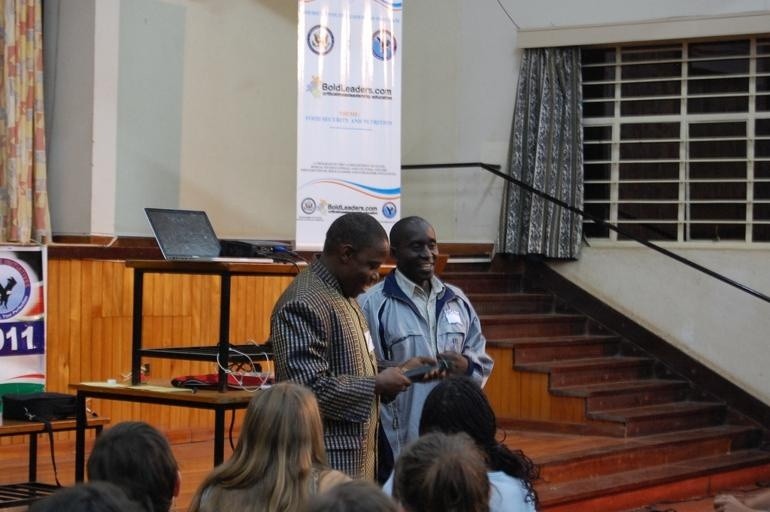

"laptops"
[144,207,274,263]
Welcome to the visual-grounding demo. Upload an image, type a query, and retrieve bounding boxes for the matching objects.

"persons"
[293,478,405,511]
[27,479,155,512]
[267,211,449,479]
[379,374,545,512]
[187,381,353,512]
[86,419,182,511]
[355,215,496,488]
[712,489,770,512]
[392,431,494,511]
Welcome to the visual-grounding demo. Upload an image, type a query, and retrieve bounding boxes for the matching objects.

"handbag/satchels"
[1,392,103,488]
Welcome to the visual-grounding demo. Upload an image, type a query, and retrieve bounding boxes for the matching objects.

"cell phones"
[404,366,432,382]
